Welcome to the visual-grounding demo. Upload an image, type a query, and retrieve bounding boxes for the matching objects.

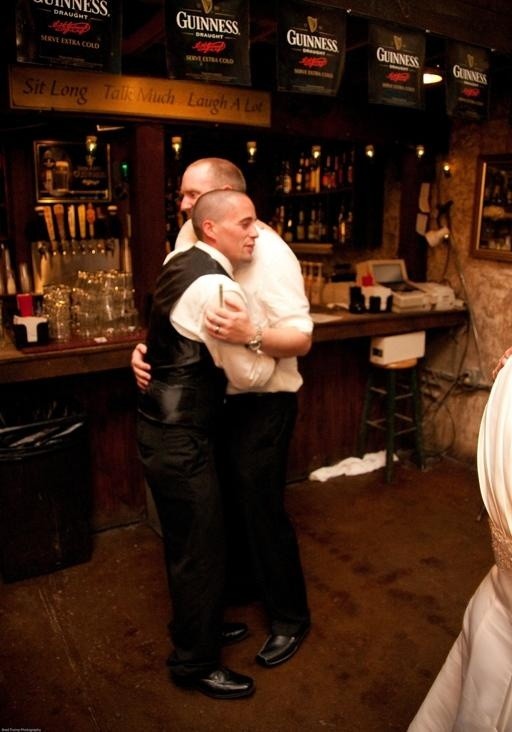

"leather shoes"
[197,665,256,700]
[221,622,249,645]
[256,626,312,669]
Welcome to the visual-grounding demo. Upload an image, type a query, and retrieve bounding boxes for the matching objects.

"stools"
[355,359,424,485]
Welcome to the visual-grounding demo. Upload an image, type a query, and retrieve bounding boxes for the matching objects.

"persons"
[139,189,276,697]
[408,342,511,731]
[130,157,314,668]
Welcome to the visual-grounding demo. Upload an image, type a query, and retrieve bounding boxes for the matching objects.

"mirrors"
[469,151,512,263]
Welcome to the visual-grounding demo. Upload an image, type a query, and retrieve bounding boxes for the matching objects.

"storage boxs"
[425,42,492,121]
[0,0,123,71]
[343,19,423,110]
[123,0,252,91]
[252,7,348,98]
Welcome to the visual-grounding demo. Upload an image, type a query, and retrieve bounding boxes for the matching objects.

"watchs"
[245,326,262,352]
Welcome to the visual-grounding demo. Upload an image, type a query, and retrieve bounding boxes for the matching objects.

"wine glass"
[300,259,324,306]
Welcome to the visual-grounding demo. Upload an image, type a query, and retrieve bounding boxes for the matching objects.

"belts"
[223,390,266,404]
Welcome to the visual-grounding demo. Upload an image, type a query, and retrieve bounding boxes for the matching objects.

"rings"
[214,326,220,335]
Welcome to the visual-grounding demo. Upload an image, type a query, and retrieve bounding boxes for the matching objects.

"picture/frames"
[32,139,112,205]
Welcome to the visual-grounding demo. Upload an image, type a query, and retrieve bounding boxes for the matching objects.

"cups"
[42,270,137,339]
